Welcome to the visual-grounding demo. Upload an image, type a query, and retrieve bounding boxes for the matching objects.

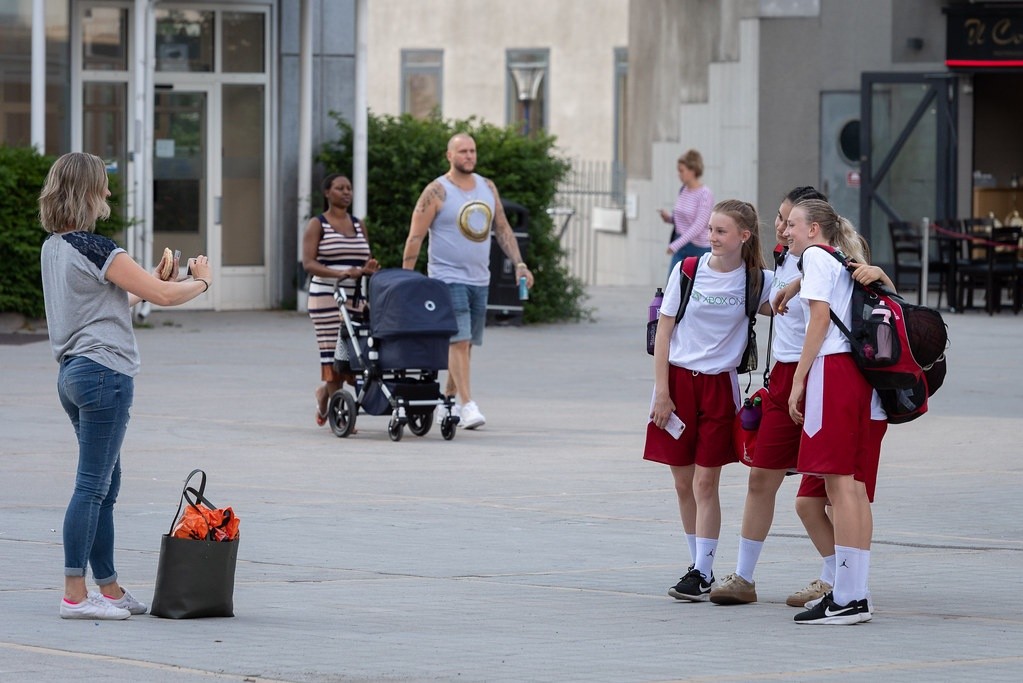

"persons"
[649,200,774,601]
[660,149,715,286]
[39,152,213,619]
[708,185,835,606]
[303,174,381,433]
[805,236,898,615]
[774,200,872,625]
[401,133,534,430]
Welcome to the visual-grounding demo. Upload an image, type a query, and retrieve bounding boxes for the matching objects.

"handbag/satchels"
[150,469,240,619]
[732,387,771,467]
[333,273,367,374]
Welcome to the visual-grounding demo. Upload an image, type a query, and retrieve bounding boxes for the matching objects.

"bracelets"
[195,279,208,292]
[517,263,527,268]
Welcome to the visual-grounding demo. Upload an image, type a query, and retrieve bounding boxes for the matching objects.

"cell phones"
[186,258,197,276]
[665,412,686,440]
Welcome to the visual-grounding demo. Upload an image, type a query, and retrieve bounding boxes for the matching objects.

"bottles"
[870,300,891,324]
[741,397,762,430]
[647,288,664,354]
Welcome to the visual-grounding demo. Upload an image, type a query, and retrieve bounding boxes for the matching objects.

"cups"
[519,276,528,300]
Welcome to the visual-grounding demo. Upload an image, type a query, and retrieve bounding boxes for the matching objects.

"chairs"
[888,217,1023,315]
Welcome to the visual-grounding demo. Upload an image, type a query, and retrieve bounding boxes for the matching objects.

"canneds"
[518,276,529,300]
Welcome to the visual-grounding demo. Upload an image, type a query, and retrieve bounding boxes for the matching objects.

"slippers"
[339,422,358,434]
[314,389,329,426]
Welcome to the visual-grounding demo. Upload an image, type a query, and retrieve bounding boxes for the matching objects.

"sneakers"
[60,591,130,620]
[786,578,833,607]
[668,562,716,602]
[794,591,872,625]
[710,572,757,605]
[461,401,485,429]
[436,400,463,426]
[804,588,873,613]
[101,586,147,614]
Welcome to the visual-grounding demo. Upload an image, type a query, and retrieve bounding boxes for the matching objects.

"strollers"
[327,267,461,442]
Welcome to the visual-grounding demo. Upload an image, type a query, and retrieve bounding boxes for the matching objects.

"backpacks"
[798,244,947,424]
